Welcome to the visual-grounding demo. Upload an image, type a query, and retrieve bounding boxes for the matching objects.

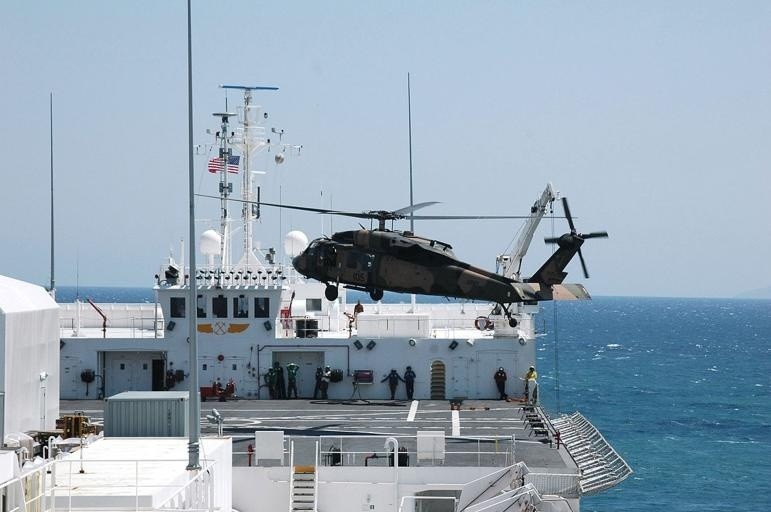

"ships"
[0,0,634,512]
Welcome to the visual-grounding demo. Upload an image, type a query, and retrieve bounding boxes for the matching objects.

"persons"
[353,300,363,329]
[525,366,537,398]
[494,367,508,400]
[381,369,405,399]
[264,361,331,399]
[404,366,416,401]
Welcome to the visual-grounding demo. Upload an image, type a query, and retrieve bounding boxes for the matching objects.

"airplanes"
[194,182,609,328]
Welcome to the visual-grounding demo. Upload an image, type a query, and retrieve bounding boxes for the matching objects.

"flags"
[208,155,239,175]
